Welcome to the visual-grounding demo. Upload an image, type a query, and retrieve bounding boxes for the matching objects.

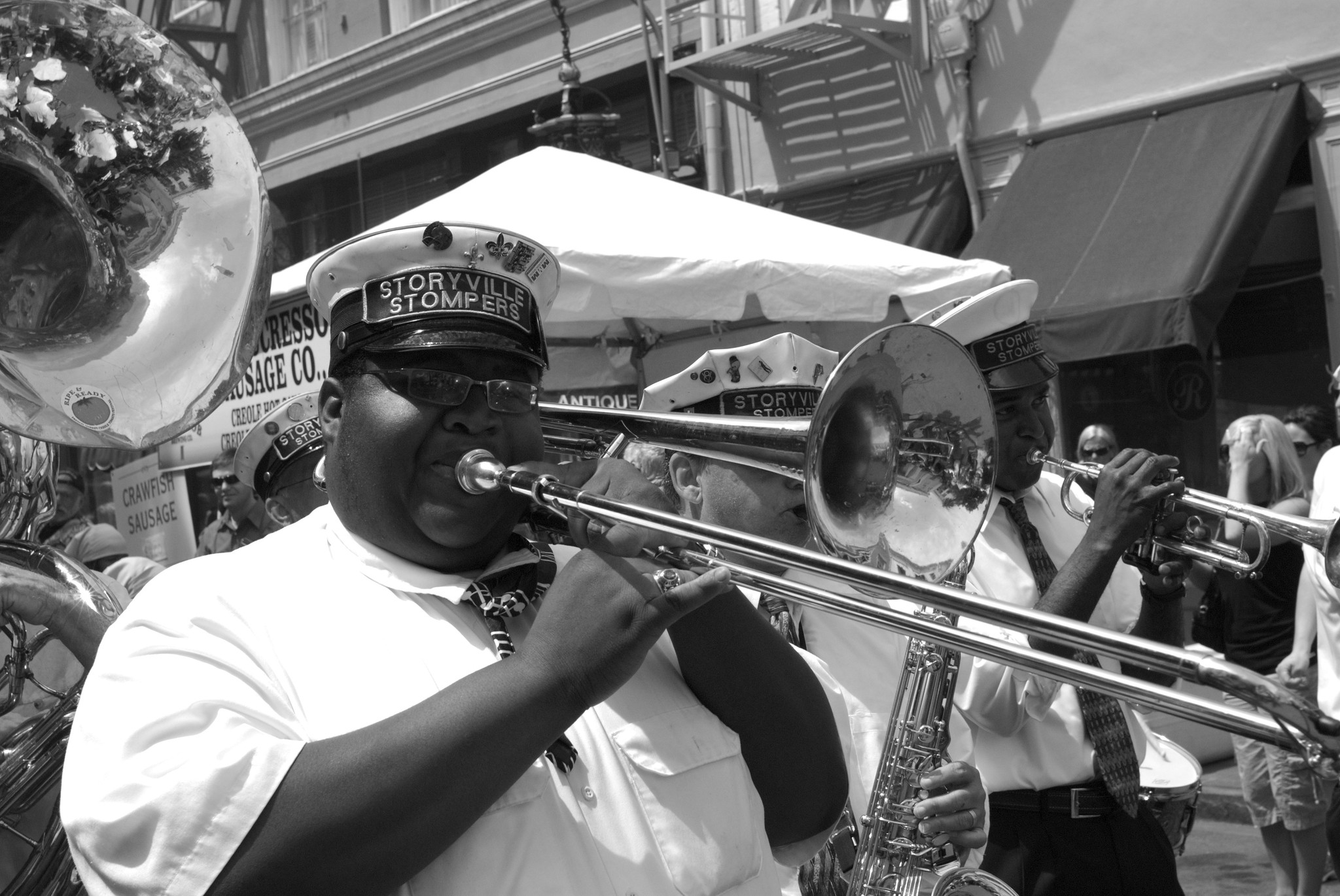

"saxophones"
[787,500,1024,895]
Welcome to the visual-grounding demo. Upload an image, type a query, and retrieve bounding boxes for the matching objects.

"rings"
[969,809,978,830]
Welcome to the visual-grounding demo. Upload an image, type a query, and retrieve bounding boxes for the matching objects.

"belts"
[989,780,1113,819]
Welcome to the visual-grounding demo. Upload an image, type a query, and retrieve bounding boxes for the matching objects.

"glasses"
[212,474,239,487]
[1334,390,1340,400]
[1293,439,1326,457]
[1081,444,1115,458]
[330,369,545,414]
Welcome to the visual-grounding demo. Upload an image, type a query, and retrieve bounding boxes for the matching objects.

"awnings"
[950,80,1312,359]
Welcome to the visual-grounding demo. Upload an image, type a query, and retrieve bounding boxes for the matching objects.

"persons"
[922,276,1199,896]
[60,219,850,896]
[1066,362,1340,896]
[227,332,991,895]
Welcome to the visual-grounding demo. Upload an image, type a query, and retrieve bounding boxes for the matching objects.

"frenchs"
[0,3,269,895]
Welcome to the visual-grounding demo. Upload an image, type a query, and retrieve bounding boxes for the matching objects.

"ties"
[461,530,579,772]
[999,497,1140,819]
[759,592,801,647]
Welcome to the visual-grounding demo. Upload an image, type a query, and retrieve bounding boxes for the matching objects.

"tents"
[269,145,1016,405]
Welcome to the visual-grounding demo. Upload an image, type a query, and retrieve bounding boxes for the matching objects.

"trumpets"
[1032,447,1339,610]
[529,323,997,583]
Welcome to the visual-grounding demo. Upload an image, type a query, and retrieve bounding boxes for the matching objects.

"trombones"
[457,453,1340,895]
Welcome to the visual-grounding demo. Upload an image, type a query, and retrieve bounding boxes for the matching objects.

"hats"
[233,390,326,502]
[908,295,973,325]
[930,278,1058,390]
[638,332,839,482]
[58,467,85,494]
[306,221,561,377]
